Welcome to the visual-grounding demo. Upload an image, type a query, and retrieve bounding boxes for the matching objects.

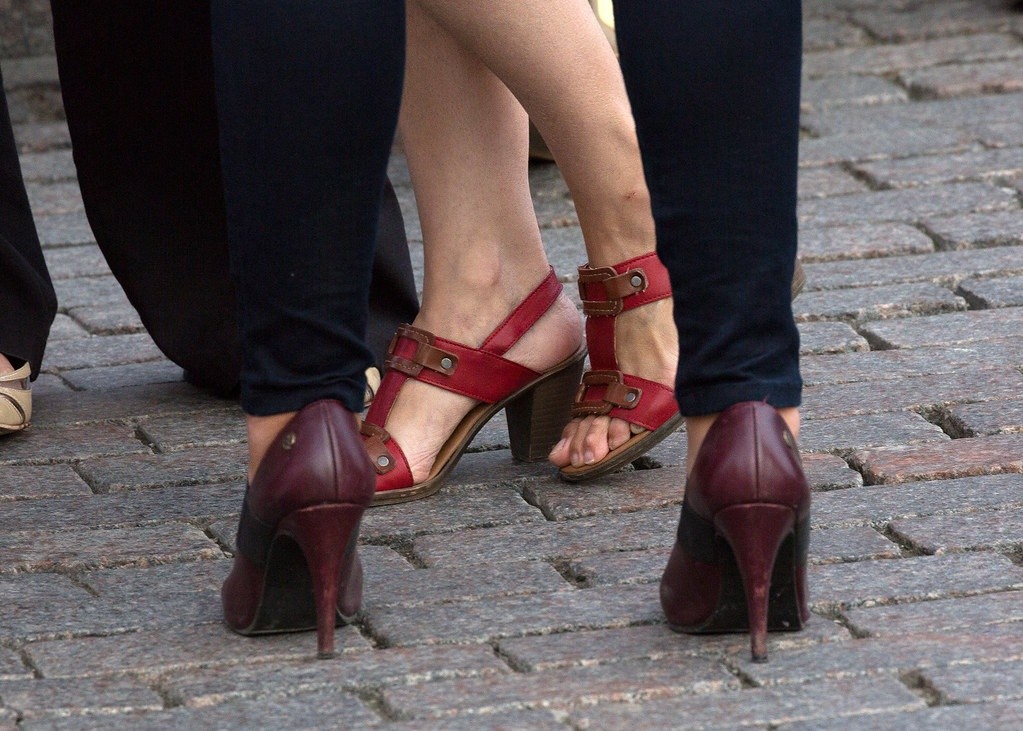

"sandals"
[0,361,32,434]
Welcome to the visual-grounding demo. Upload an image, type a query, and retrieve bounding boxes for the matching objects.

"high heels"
[220,399,377,661]
[660,401,812,662]
[559,251,808,482]
[362,263,588,508]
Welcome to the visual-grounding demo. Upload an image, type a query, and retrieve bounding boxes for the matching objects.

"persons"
[0,0,813,662]
